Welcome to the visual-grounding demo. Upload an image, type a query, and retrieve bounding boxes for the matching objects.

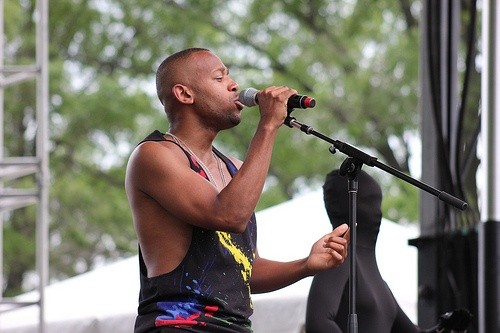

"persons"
[124,47,350,333]
[307,170,424,333]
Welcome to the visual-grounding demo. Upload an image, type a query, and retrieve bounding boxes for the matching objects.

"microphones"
[239,87,316,109]
